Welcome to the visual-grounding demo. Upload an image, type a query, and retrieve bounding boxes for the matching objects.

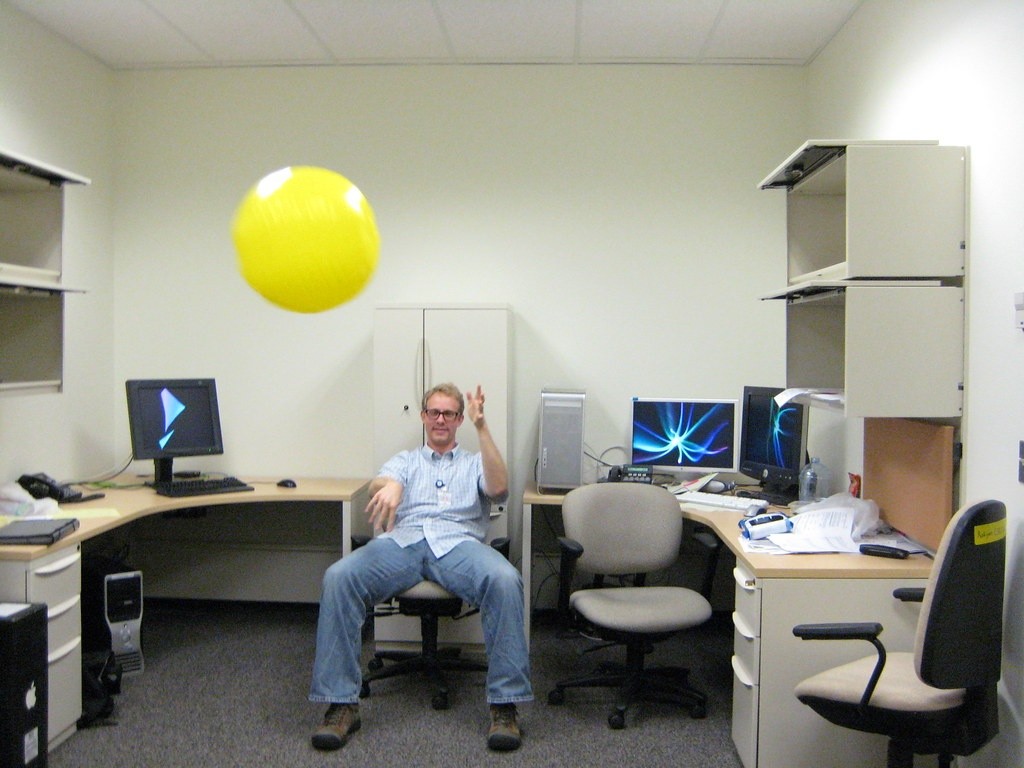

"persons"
[308,382,534,755]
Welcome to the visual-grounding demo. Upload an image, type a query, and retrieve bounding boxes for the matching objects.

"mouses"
[743,504,767,516]
[277,479,297,488]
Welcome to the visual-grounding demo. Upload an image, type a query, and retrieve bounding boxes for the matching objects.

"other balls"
[233,164,382,316]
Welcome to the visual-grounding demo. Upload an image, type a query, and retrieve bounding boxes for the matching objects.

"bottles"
[799,457,833,505]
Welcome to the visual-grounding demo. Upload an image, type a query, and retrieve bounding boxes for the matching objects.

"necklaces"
[422,446,455,488]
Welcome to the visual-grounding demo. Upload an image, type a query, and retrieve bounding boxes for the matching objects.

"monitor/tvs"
[739,386,811,505]
[126,378,224,486]
[630,398,741,493]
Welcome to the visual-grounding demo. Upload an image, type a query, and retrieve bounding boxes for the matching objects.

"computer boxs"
[536,387,586,495]
[0,601,49,768]
[79,554,144,679]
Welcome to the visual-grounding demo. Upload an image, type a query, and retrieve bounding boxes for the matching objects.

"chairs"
[352,537,510,710]
[546,482,713,728]
[792,501,1007,768]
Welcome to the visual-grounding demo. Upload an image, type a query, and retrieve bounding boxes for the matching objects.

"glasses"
[425,409,460,423]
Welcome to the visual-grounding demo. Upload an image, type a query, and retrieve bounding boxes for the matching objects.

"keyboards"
[155,477,255,498]
[675,491,769,511]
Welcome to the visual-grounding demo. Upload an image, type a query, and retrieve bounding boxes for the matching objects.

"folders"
[0,516,80,545]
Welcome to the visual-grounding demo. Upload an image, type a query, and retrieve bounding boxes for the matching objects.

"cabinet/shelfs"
[0,150,90,398]
[786,144,967,417]
[374,307,514,549]
[521,481,937,768]
[0,473,372,768]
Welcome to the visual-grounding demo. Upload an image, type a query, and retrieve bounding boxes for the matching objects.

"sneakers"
[311,703,361,750]
[488,702,522,751]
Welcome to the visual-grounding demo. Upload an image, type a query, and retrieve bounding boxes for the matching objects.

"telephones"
[606,462,654,483]
[17,472,82,503]
